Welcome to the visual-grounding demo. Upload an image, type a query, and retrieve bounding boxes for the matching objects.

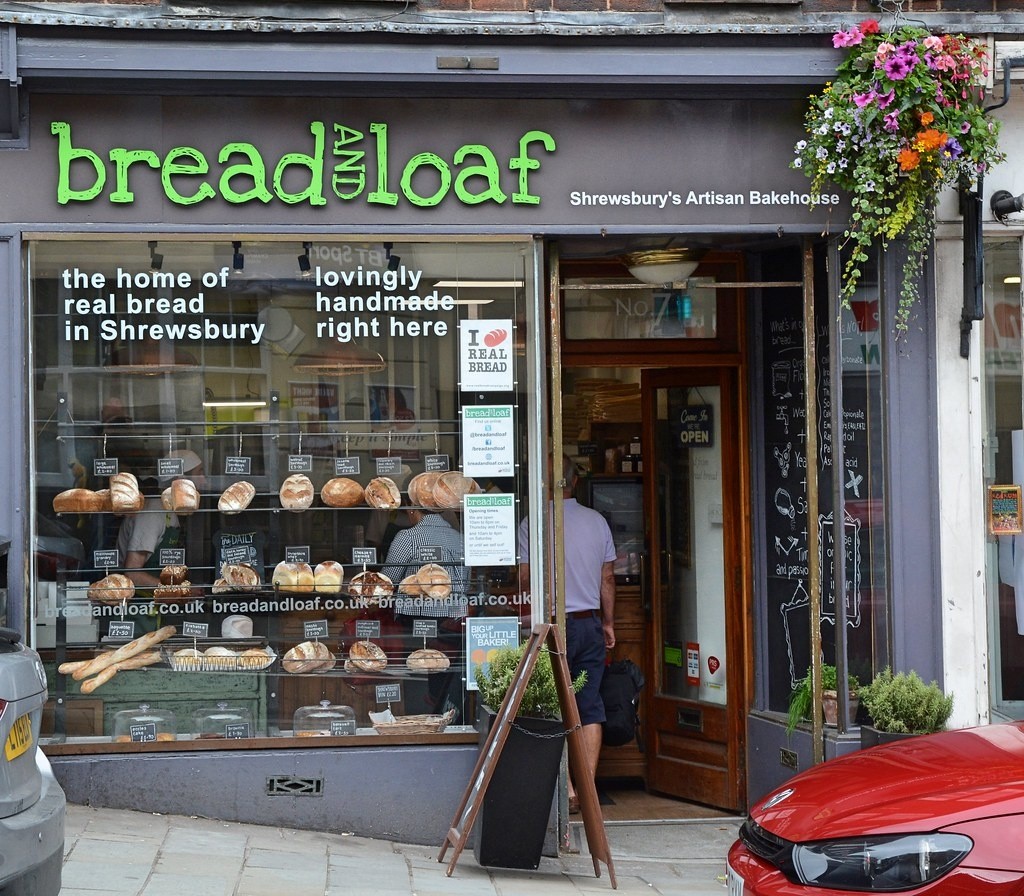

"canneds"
[620,436,644,473]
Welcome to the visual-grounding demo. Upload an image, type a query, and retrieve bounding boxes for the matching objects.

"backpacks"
[601,648,646,756]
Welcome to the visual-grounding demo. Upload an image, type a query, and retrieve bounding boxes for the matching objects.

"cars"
[719,717,1024,896]
[0,622,66,895]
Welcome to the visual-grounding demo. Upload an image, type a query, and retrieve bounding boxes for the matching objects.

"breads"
[58,625,451,695]
[115,732,175,742]
[53,470,481,599]
[297,731,319,736]
[198,732,223,739]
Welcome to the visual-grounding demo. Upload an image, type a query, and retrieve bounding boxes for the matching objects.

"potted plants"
[469,634,593,871]
[856,662,954,748]
[784,663,872,736]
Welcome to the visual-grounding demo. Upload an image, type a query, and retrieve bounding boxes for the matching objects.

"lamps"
[383,242,401,271]
[202,395,270,409]
[625,245,702,286]
[148,241,164,270]
[509,290,526,356]
[296,241,313,271]
[293,310,389,375]
[231,241,245,269]
[102,334,202,380]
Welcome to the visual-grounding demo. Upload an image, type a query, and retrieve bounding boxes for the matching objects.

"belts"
[549,607,603,623]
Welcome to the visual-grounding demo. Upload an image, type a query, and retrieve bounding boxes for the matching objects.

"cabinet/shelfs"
[32,472,523,742]
[593,590,649,785]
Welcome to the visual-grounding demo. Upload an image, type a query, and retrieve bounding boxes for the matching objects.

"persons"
[117,449,204,599]
[84,460,160,582]
[211,510,266,623]
[76,396,154,475]
[517,452,615,814]
[345,395,375,431]
[360,471,472,714]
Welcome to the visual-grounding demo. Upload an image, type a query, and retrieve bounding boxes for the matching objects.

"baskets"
[373,713,452,735]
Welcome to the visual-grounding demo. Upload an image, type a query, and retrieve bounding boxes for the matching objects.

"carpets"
[598,789,616,805]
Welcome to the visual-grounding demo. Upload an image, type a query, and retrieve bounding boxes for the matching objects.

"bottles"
[634,455,643,472]
[621,455,634,472]
[676,293,692,321]
[628,436,641,455]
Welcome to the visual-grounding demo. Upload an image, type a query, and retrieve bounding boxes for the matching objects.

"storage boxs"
[41,657,270,735]
[630,436,642,454]
[637,457,644,472]
[620,454,636,473]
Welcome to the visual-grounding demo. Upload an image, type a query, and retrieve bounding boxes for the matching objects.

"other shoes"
[568,795,580,813]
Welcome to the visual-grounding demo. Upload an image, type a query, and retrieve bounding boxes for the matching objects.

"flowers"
[789,15,1001,357]
